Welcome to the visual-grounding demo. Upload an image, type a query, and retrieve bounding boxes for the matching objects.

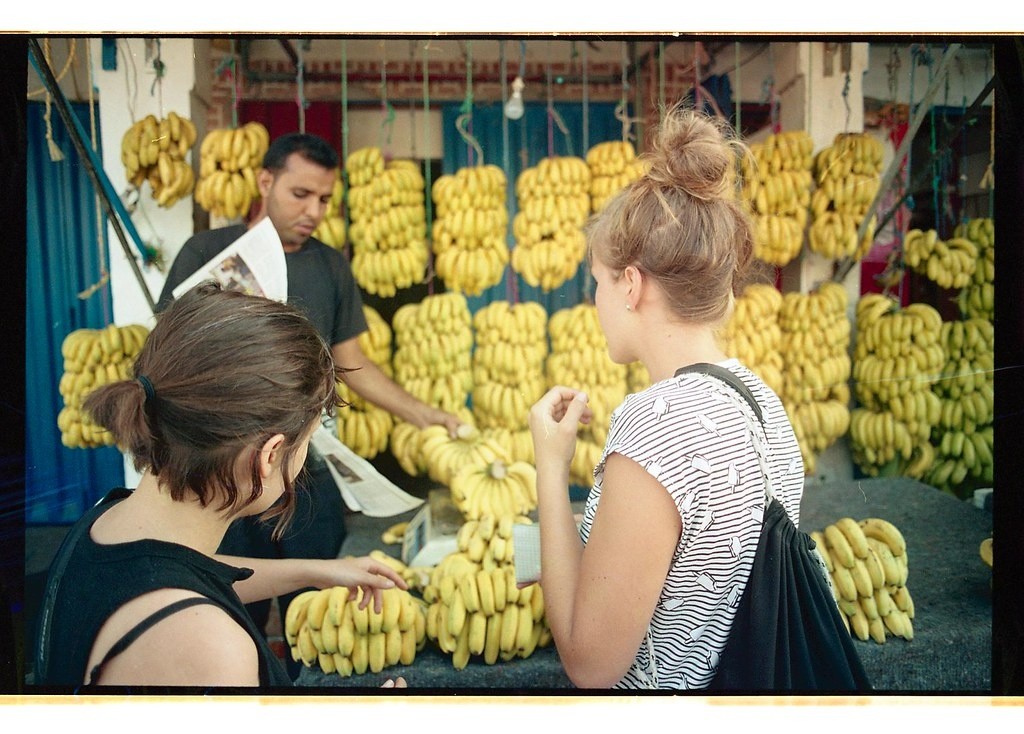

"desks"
[293,475,992,691]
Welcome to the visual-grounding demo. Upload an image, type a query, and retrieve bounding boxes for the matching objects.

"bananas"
[59,114,994,676]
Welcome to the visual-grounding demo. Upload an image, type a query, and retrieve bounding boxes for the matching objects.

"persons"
[157,134,462,685]
[530,111,804,689]
[28,279,409,686]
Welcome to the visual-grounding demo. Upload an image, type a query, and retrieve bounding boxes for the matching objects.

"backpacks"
[704,499,875,696]
